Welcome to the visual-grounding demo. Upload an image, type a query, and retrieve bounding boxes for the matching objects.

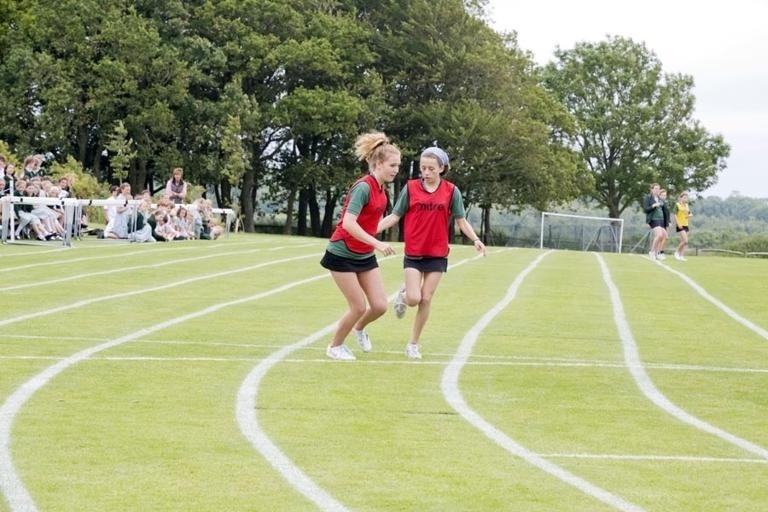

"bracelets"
[471,238,480,243]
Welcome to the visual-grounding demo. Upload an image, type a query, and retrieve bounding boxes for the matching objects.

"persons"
[643,183,664,260]
[320,130,396,361]
[658,189,671,260]
[376,147,486,359]
[0,153,224,242]
[673,193,693,261]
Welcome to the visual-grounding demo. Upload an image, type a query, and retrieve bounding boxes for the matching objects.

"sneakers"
[395,283,406,318]
[353,327,371,352]
[405,342,423,359]
[36,232,63,241]
[650,249,686,261]
[327,344,357,361]
